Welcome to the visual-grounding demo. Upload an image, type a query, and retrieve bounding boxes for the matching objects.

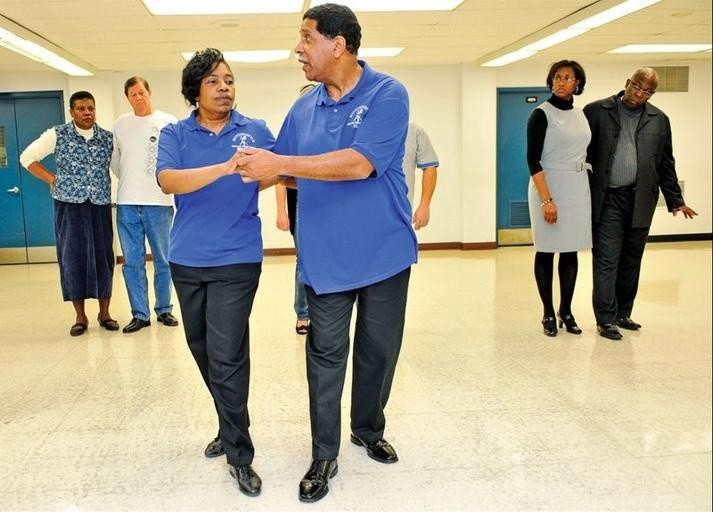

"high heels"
[557,312,582,334]
[542,316,557,336]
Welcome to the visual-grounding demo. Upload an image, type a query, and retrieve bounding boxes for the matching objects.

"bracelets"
[539,198,552,207]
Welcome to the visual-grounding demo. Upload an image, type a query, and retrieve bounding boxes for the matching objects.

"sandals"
[296,320,308,334]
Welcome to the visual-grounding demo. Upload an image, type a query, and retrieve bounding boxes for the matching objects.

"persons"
[582,67,698,341]
[526,61,594,337]
[402,122,439,229]
[153,48,298,497]
[19,90,119,336]
[238,2,418,502]
[273,85,323,334]
[109,77,179,334]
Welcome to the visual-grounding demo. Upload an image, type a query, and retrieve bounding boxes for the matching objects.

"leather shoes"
[123,318,150,333]
[300,458,338,503]
[205,439,226,458]
[230,463,261,496]
[351,428,397,463]
[597,323,621,340]
[157,313,178,326]
[98,313,119,330]
[616,317,641,329]
[70,319,87,336]
